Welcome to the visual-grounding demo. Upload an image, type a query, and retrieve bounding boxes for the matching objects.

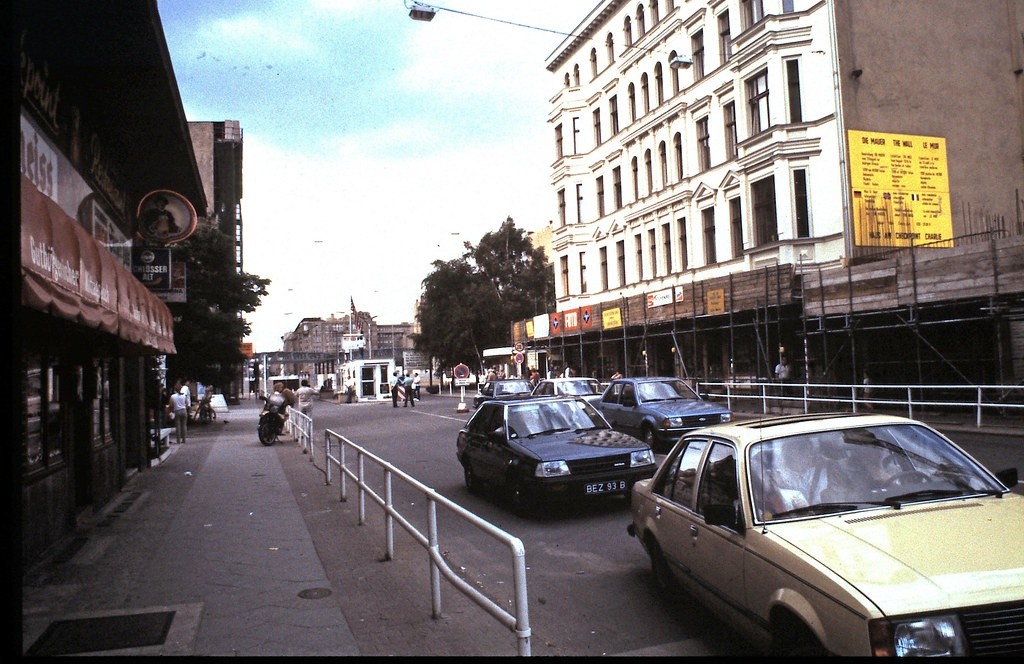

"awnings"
[18,174,178,357]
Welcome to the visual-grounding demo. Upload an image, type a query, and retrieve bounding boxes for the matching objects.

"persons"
[752,431,882,513]
[140,195,182,239]
[295,379,319,441]
[560,366,575,378]
[611,371,623,395]
[775,357,792,399]
[390,372,399,408]
[530,368,540,386]
[862,371,874,412]
[401,374,415,407]
[478,369,506,383]
[160,378,191,444]
[413,373,420,401]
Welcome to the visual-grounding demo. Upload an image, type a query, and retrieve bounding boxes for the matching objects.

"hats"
[488,369,493,372]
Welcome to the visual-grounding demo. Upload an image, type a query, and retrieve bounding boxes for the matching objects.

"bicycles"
[199,395,216,424]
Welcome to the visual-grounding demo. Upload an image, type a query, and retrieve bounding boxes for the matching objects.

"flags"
[350,299,363,330]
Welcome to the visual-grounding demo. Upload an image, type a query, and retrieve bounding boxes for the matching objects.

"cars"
[471,379,535,408]
[626,411,1024,656]
[529,376,606,409]
[454,395,661,515]
[593,377,732,452]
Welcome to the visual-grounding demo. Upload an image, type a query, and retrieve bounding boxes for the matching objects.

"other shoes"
[413,397,419,401]
[403,405,407,407]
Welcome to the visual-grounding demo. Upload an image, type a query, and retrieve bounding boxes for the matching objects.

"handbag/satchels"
[411,382,416,389]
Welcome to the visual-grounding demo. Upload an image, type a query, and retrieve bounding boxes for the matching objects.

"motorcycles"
[257,395,290,446]
